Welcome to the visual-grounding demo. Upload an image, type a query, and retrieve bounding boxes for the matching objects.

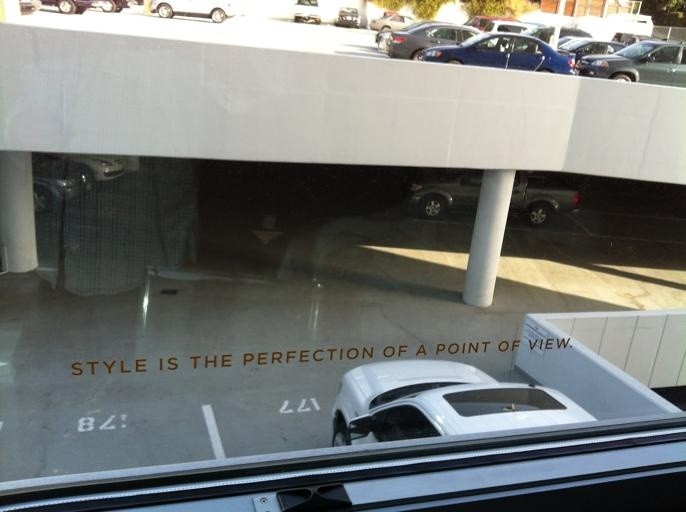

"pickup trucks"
[407,170,583,230]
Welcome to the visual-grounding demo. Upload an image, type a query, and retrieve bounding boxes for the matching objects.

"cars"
[31,150,130,213]
[20,0,685,90]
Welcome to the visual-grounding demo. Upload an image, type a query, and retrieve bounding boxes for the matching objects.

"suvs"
[333,357,600,451]
